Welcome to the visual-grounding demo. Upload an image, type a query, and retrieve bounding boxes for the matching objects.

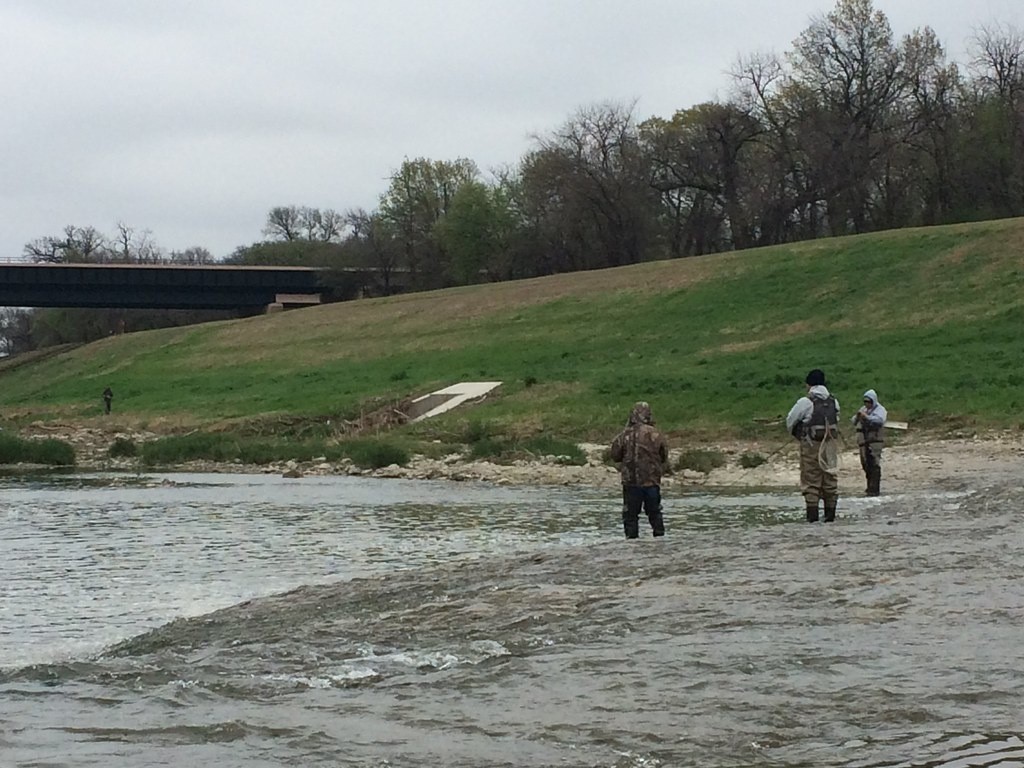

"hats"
[805,369,824,385]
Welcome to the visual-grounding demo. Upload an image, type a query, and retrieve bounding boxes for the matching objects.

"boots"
[824,507,835,523]
[806,506,819,522]
[865,466,881,497]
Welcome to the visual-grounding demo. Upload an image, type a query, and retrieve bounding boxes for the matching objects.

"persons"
[610,402,674,540]
[786,369,841,524]
[849,389,888,498]
[103,387,113,414]
